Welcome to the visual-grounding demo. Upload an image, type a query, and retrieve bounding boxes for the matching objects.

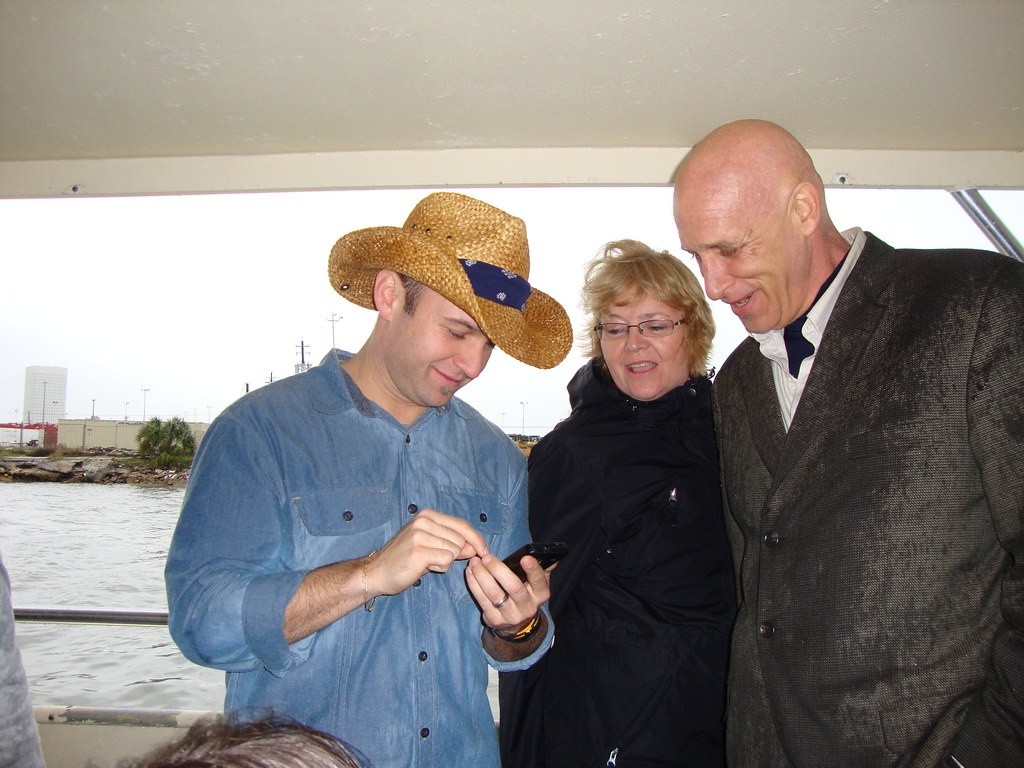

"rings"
[495,592,509,608]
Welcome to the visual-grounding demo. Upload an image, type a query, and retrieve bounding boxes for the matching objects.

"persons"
[165,192,574,768]
[498,239,738,768]
[673,119,1024,768]
[0,556,371,768]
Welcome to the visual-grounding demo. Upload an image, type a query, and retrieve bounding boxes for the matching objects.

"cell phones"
[500,541,571,583]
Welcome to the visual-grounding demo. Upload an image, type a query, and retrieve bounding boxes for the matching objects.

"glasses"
[595,316,689,341]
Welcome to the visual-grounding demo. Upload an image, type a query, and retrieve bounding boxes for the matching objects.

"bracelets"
[362,551,377,611]
[495,610,540,643]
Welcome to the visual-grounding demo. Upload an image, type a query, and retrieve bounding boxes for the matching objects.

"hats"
[328,191,574,369]
